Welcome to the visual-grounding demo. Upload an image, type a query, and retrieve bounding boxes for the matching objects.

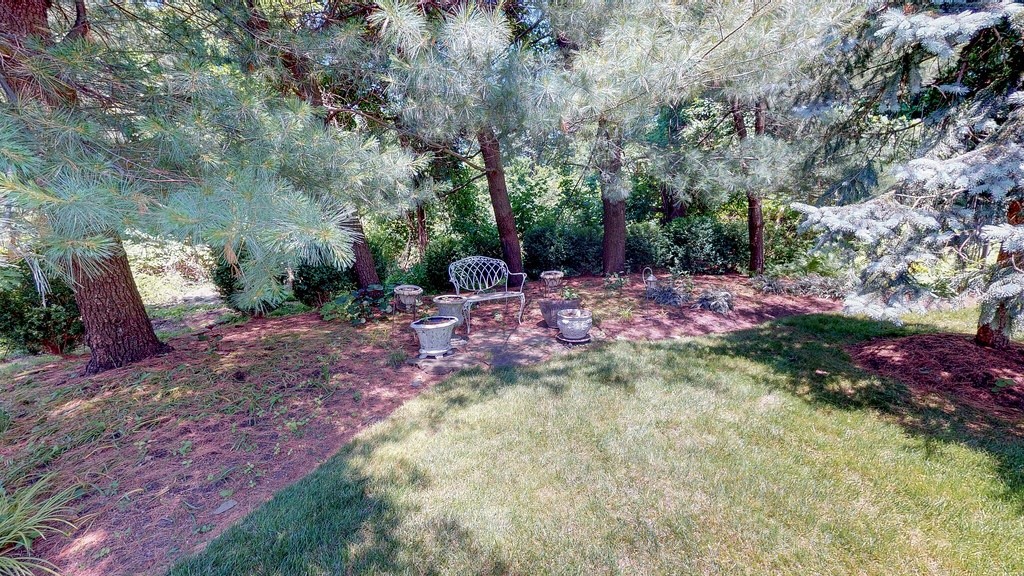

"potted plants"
[537,286,583,329]
[410,315,458,359]
[433,294,467,327]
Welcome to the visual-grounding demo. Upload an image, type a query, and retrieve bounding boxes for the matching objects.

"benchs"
[448,255,527,334]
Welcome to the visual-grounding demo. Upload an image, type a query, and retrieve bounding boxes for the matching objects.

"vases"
[541,270,564,288]
[556,309,593,340]
[394,285,423,304]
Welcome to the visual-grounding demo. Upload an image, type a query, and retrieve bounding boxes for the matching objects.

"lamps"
[646,275,658,289]
